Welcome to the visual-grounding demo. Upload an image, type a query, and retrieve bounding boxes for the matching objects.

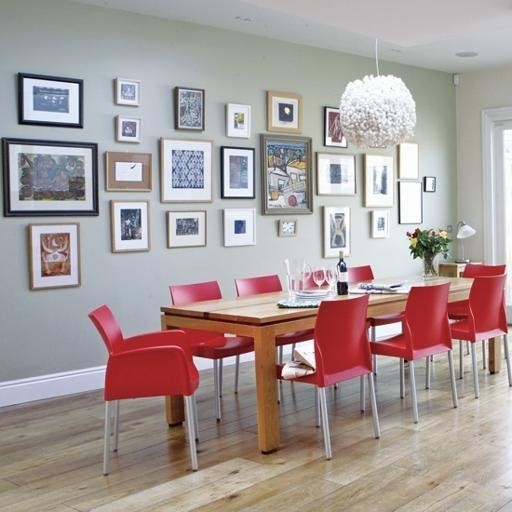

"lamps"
[339,37,417,150]
[455,220,476,263]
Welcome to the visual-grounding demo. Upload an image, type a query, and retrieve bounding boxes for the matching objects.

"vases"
[423,258,437,280]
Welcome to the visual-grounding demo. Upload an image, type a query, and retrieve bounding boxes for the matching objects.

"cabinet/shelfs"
[438,261,481,278]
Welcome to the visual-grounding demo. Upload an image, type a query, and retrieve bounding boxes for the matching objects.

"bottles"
[337,251,348,295]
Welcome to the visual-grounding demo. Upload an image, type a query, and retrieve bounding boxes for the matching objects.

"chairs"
[87,305,200,476]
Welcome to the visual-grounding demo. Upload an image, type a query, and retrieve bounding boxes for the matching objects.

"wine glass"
[313,266,326,289]
[326,269,337,291]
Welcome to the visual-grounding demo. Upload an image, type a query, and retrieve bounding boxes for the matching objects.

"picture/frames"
[174,87,205,132]
[159,137,214,203]
[28,223,82,290]
[314,107,437,259]
[221,91,313,248]
[17,72,85,128]
[105,150,154,192]
[1,137,99,217]
[110,199,152,253]
[112,77,142,107]
[166,210,207,248]
[115,115,144,144]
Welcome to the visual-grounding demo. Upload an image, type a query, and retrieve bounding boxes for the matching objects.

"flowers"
[406,224,452,275]
